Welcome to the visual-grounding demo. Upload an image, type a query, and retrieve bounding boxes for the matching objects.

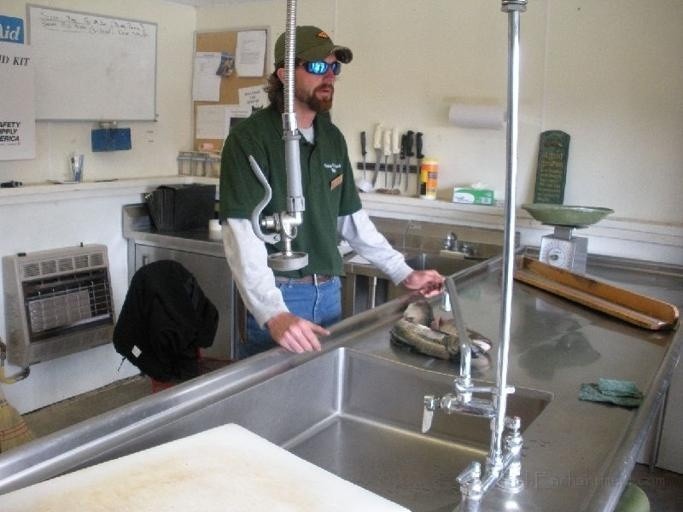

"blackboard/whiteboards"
[26,3,157,122]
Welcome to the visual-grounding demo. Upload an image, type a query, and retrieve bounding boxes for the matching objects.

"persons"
[220,26,445,357]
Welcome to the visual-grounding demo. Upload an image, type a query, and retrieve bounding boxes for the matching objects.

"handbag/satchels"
[143,182,216,231]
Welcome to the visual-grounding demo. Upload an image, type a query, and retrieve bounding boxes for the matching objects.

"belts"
[275,273,334,284]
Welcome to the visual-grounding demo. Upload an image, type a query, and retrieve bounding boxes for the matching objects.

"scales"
[521,204,615,277]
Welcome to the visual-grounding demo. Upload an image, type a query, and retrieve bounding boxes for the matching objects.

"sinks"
[0,347,555,512]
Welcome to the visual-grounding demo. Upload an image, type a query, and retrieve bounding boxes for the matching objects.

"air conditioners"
[0,242,118,370]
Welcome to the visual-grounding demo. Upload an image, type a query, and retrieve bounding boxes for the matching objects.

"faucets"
[421,277,496,435]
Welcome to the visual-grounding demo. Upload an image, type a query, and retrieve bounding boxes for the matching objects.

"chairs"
[129,256,246,395]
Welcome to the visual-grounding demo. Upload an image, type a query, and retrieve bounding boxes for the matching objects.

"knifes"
[358,123,424,192]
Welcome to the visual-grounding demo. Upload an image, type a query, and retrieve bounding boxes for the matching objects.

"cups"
[207,218,223,241]
[68,153,83,182]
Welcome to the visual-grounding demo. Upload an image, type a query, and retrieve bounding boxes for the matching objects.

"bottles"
[416,156,439,200]
[177,151,221,178]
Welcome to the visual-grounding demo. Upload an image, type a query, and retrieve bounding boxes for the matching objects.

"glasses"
[295,61,342,74]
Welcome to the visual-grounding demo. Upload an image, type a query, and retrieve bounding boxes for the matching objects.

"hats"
[273,25,353,66]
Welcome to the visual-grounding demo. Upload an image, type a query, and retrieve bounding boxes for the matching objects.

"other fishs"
[402,298,434,327]
[388,316,485,361]
[434,317,493,354]
